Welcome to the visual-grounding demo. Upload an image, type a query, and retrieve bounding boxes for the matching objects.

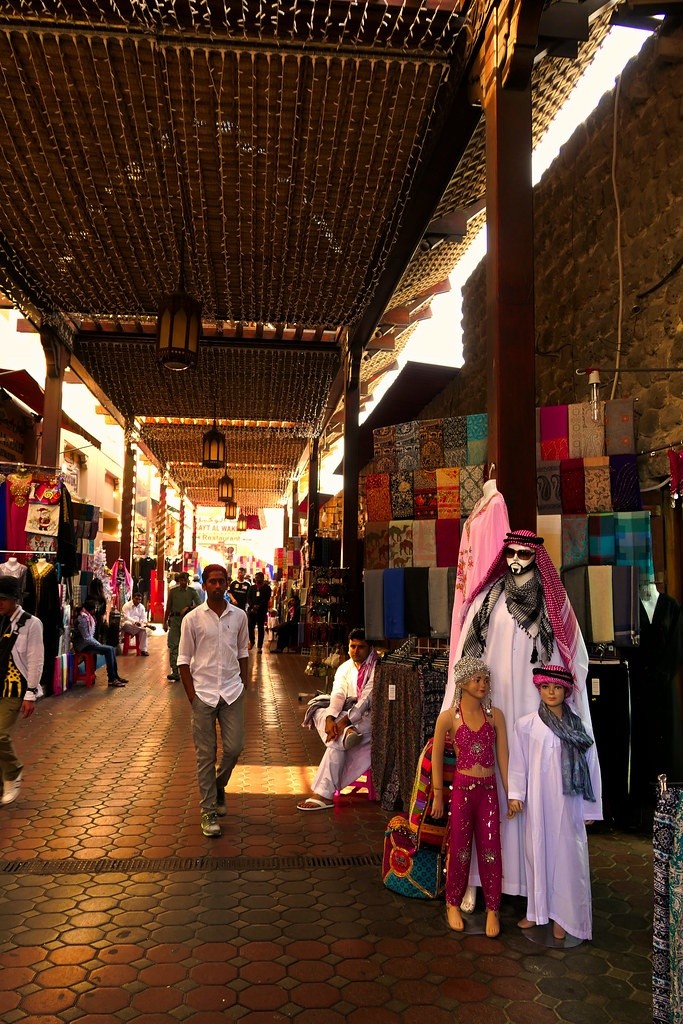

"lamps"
[237,508,247,531]
[218,467,234,502]
[202,402,226,469]
[156,228,202,371]
[225,502,237,519]
[31,412,43,424]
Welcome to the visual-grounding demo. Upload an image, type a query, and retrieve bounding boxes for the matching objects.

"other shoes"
[148,625,156,630]
[118,678,129,682]
[270,649,282,653]
[257,650,262,654]
[248,641,254,650]
[167,675,180,681]
[108,680,125,687]
[141,651,149,656]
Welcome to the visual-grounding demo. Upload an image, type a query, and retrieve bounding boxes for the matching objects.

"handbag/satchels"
[381,815,446,898]
[408,735,456,846]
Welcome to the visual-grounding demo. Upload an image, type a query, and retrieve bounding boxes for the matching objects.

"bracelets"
[188,606,191,610]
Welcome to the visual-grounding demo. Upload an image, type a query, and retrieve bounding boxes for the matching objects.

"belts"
[172,612,181,616]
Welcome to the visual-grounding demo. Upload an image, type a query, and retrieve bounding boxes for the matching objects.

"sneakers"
[1,771,22,804]
[200,812,221,836]
[217,792,227,815]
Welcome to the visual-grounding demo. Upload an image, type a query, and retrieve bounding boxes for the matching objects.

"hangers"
[377,640,450,670]
[588,642,627,664]
[655,774,683,795]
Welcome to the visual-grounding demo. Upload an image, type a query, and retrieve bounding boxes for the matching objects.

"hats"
[452,657,493,718]
[532,665,574,698]
[177,572,189,579]
[193,574,200,580]
[0,576,29,599]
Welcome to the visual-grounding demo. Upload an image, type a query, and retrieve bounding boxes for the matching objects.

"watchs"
[26,688,38,694]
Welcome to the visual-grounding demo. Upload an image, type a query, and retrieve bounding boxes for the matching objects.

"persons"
[85,578,107,641]
[460,530,603,915]
[168,572,253,613]
[120,592,157,657]
[163,572,202,681]
[295,628,383,811]
[72,600,129,687]
[0,575,45,805]
[440,479,512,713]
[506,665,593,944]
[0,556,35,617]
[109,557,135,612]
[606,574,680,833]
[246,572,272,654]
[227,568,251,611]
[428,656,512,937]
[177,564,249,835]
[270,595,301,654]
[27,558,65,698]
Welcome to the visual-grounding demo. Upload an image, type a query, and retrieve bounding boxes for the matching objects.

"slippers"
[296,798,334,810]
[344,731,363,749]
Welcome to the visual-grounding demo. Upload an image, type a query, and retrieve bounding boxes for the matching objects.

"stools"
[74,652,96,688]
[123,631,142,656]
[334,768,376,801]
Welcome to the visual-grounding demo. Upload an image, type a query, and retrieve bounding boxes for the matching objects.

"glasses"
[504,548,536,560]
